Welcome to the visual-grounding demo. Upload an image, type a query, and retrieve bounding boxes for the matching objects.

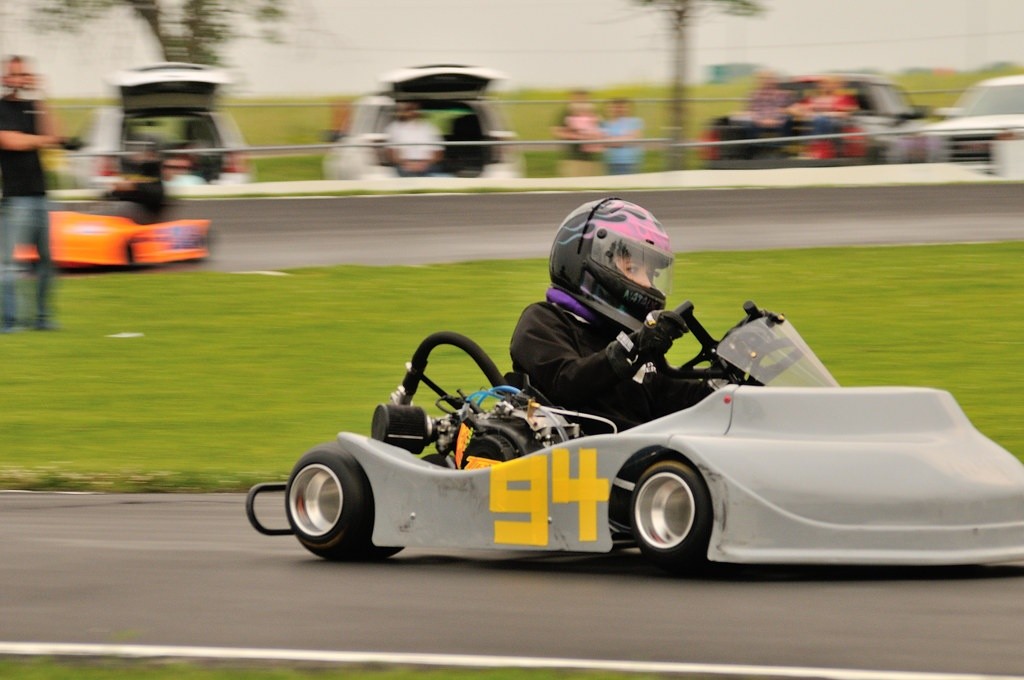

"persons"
[747,70,864,167]
[377,102,447,177]
[0,55,84,330]
[510,197,714,435]
[551,91,644,176]
[93,142,249,223]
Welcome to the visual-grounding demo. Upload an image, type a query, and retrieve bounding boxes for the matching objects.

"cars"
[321,65,527,180]
[64,62,254,188]
[701,74,1024,170]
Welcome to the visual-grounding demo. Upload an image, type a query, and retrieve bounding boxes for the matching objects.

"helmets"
[548,197,674,334]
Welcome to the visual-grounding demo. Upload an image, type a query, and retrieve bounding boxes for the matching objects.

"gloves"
[604,309,689,380]
[716,308,784,386]
